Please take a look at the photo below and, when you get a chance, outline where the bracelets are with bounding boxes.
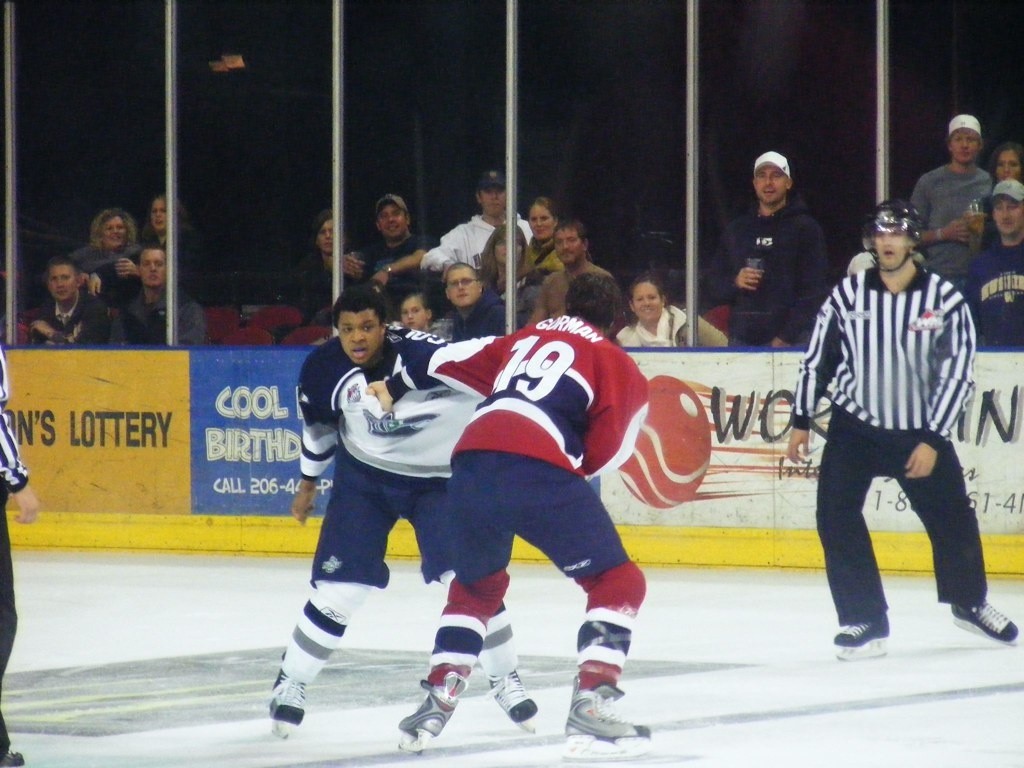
[386,263,393,281]
[936,228,944,240]
[45,329,52,338]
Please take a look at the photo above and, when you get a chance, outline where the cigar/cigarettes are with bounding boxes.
[755,263,773,274]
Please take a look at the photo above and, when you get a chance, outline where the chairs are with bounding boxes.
[202,306,243,342]
[222,326,276,347]
[247,305,304,339]
[702,305,729,334]
[280,325,332,345]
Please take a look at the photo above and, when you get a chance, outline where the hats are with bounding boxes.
[991,179,1024,202]
[376,194,409,216]
[753,150,791,179]
[477,169,507,191]
[949,114,981,136]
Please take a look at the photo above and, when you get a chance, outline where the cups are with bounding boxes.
[966,199,984,228]
[746,258,762,288]
[115,259,128,279]
[349,252,364,261]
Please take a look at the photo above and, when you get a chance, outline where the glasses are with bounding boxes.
[446,278,479,287]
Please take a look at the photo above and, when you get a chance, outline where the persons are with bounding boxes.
[29,190,209,349]
[295,171,728,349]
[0,345,39,768]
[964,178,1024,347]
[910,114,1024,296]
[261,284,538,739]
[398,272,651,761]
[788,198,1018,646]
[711,151,826,347]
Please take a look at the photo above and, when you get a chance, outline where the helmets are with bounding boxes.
[869,197,922,240]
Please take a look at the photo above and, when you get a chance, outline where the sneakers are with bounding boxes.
[834,612,890,662]
[489,670,540,734]
[560,675,653,764]
[0,750,26,768]
[950,600,1020,647]
[269,668,306,740]
[397,670,469,756]
